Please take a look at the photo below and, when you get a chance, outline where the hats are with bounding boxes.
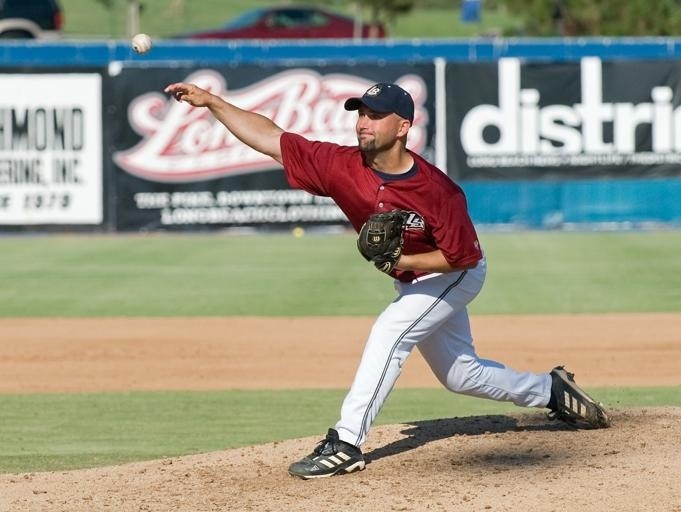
[344,82,414,120]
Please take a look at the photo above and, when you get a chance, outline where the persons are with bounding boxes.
[163,82,610,480]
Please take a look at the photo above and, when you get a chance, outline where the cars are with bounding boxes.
[0,1,64,39]
[165,5,388,39]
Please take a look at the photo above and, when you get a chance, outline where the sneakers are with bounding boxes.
[548,365,609,426]
[288,426,366,479]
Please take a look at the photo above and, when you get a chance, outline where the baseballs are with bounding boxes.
[132,34,152,54]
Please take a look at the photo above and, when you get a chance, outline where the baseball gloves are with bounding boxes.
[356,209,410,275]
